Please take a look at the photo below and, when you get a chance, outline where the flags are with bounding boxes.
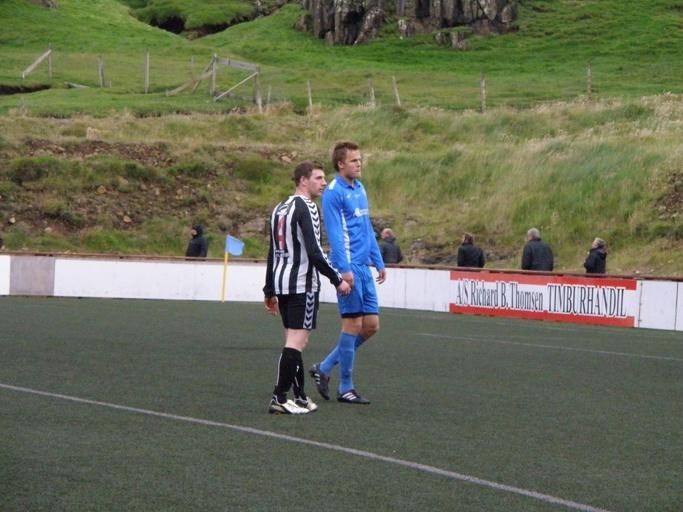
[225,235,245,257]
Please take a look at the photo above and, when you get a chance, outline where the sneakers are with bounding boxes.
[337,389,371,405]
[308,363,331,401]
[293,395,318,412]
[268,394,310,414]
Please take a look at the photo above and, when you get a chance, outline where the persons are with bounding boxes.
[309,140,386,404]
[378,228,401,264]
[459,231,485,272]
[264,160,351,414]
[584,236,606,272]
[521,227,554,271]
[184,224,208,261]
